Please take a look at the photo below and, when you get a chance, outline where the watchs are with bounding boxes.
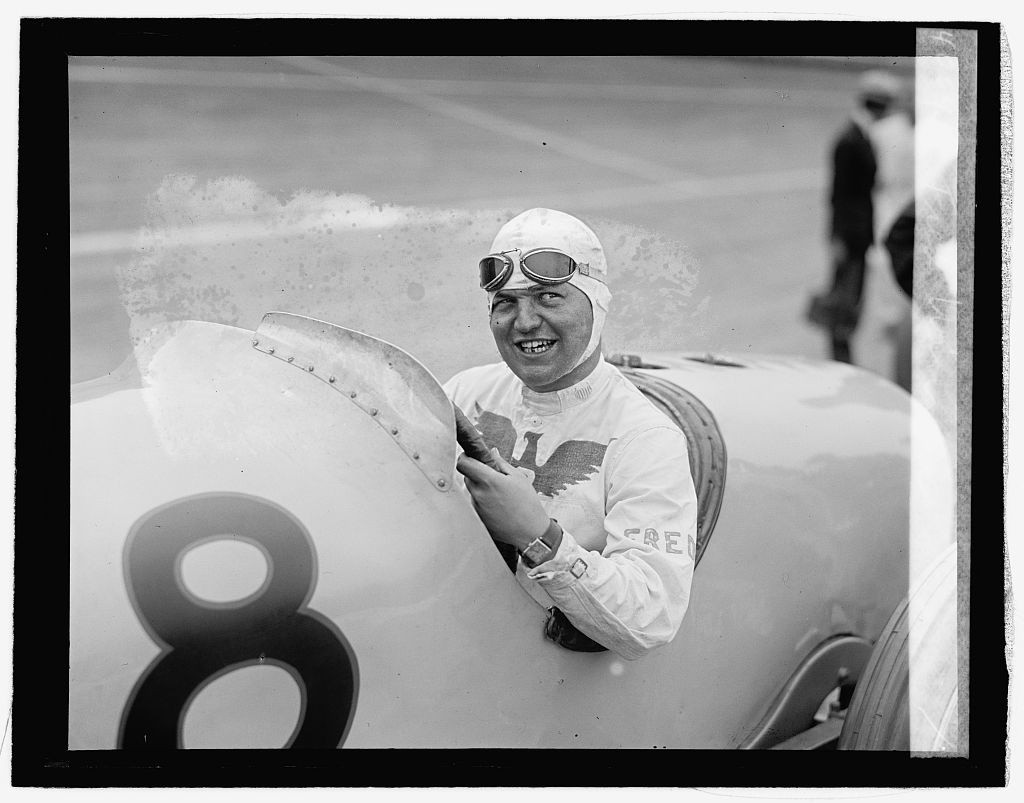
[521,519,569,575]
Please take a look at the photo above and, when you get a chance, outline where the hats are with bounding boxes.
[485,207,612,368]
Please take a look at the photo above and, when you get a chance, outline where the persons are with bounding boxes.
[809,69,896,367]
[356,208,703,659]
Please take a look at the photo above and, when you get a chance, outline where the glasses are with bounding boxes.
[479,247,606,293]
